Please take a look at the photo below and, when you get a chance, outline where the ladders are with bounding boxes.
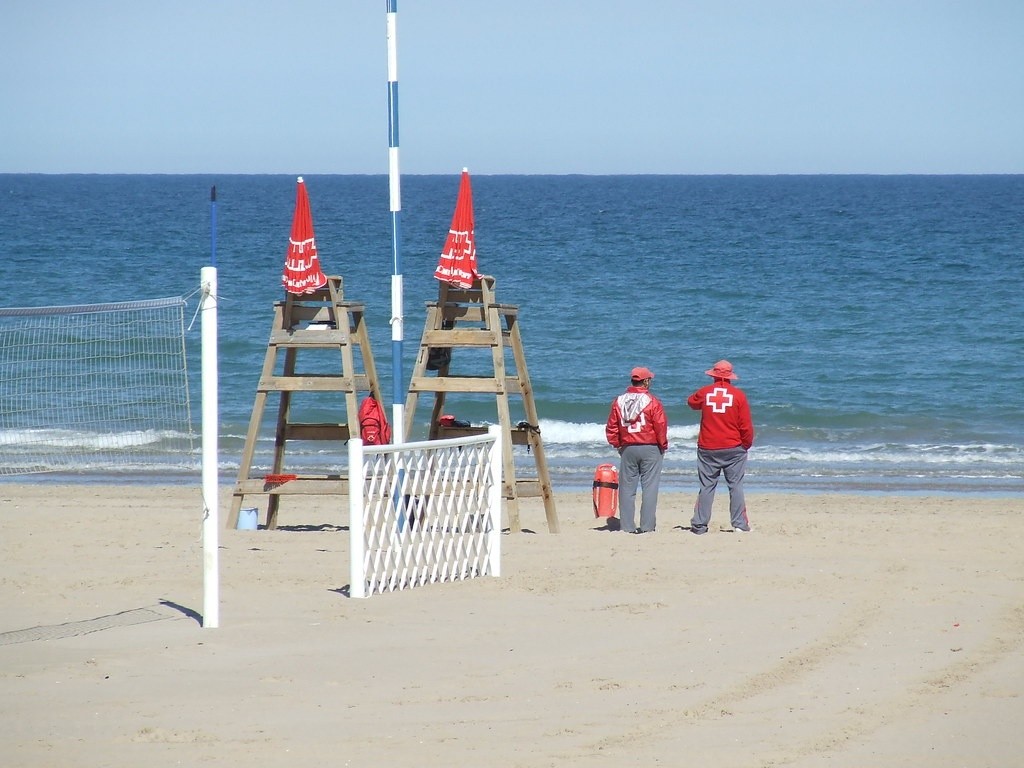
[391,302,561,544]
[225,300,402,529]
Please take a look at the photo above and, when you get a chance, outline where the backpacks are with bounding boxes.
[358,391,391,445]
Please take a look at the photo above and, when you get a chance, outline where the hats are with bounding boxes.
[631,367,654,380]
[705,360,736,379]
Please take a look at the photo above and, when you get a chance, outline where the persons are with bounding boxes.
[606,367,668,533]
[688,358,755,536]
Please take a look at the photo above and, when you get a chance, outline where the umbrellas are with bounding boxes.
[282,176,326,296]
[434,166,478,288]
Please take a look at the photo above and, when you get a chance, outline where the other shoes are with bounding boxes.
[735,528,743,532]
